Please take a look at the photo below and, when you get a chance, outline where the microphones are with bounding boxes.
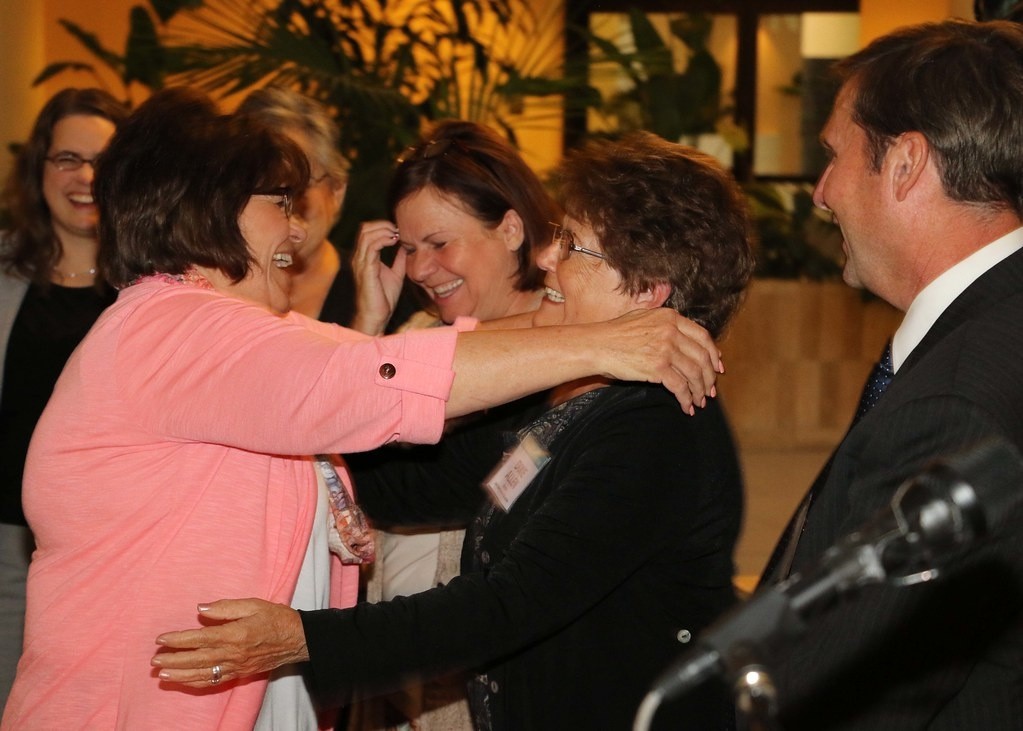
[649,435,1023,703]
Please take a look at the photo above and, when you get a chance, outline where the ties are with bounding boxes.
[787,335,894,580]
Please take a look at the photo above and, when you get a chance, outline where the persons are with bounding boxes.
[0,88,725,731]
[1,88,128,716]
[151,132,751,731]
[729,21,1023,731]
[235,84,423,731]
[351,122,562,731]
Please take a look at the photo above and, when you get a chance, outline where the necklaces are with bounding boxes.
[54,268,96,276]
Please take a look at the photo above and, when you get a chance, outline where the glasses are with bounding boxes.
[252,182,293,220]
[545,220,607,261]
[45,151,102,172]
[386,136,498,182]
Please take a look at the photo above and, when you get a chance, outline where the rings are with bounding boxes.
[212,666,221,684]
